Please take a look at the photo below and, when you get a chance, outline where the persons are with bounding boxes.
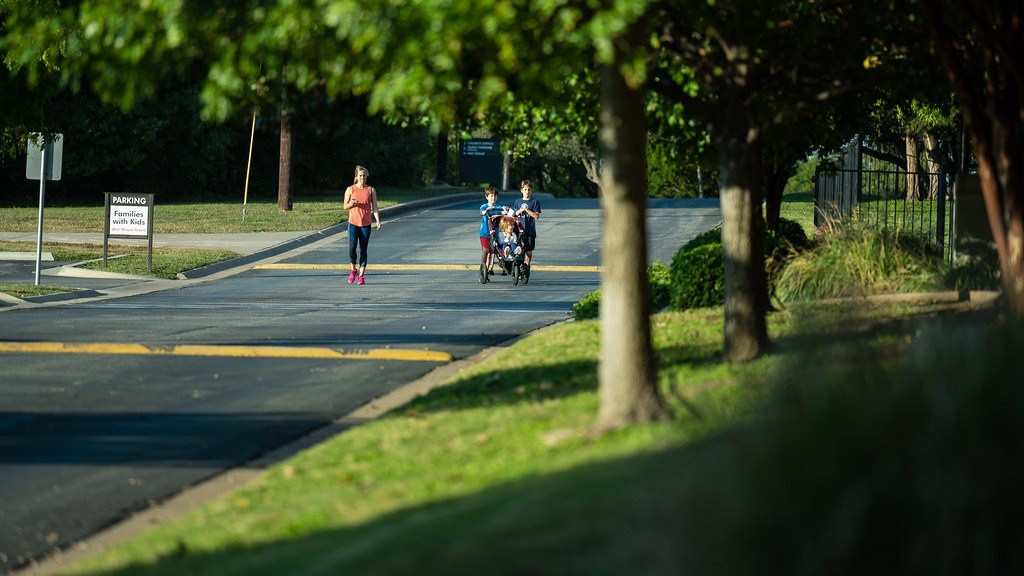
[342,165,382,284]
[475,180,542,283]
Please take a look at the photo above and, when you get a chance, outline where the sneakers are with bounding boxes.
[348,269,357,283]
[358,275,365,284]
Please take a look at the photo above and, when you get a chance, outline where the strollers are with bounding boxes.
[479,206,531,286]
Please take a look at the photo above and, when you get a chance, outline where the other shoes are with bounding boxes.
[504,246,513,260]
[488,268,494,275]
[513,246,522,259]
